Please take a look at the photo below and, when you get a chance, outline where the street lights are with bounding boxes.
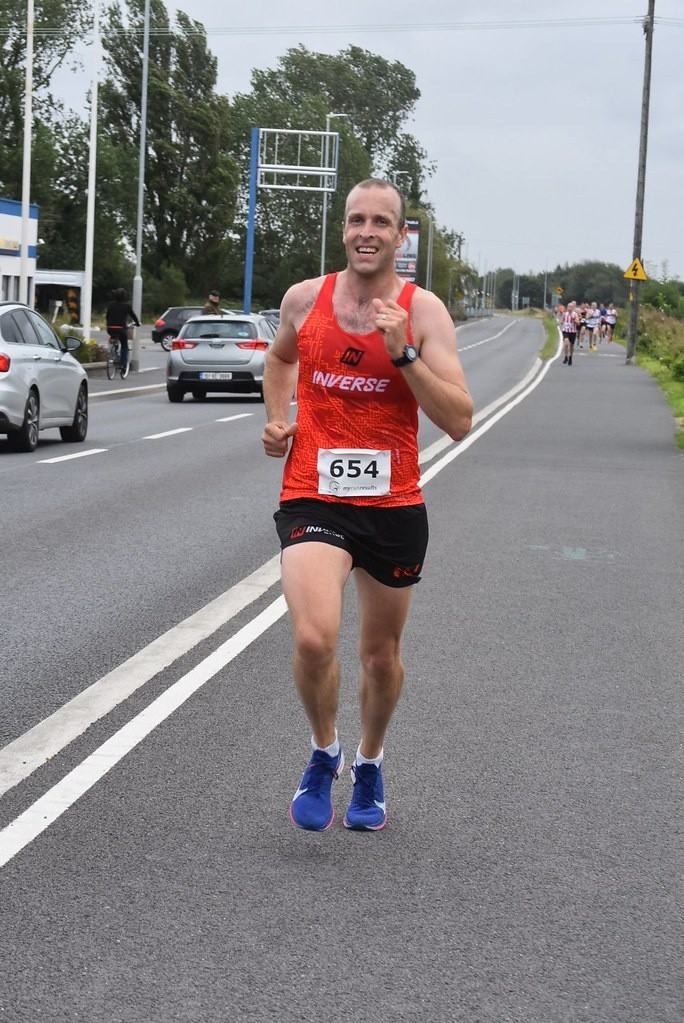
[392,169,410,185]
[319,110,354,277]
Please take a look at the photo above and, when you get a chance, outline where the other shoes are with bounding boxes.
[563,358,568,363]
[607,337,612,344]
[577,341,583,349]
[569,360,572,365]
[594,345,597,350]
[120,366,126,373]
[589,348,593,353]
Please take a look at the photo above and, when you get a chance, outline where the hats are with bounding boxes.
[209,290,220,297]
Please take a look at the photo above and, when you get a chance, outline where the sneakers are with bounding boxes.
[288,744,344,832]
[342,759,387,831]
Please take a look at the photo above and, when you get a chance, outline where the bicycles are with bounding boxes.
[106,323,141,381]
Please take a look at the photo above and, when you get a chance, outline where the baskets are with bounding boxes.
[126,326,136,340]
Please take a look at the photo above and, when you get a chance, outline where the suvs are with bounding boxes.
[0,300,90,454]
[150,305,240,352]
[164,315,296,403]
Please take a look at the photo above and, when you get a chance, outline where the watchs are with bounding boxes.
[391,344,417,367]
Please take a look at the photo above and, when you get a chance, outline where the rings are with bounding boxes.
[383,315,386,319]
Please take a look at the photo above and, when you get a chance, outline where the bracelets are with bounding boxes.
[573,318,575,320]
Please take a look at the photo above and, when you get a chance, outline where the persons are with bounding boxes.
[261,178,474,834]
[203,291,222,316]
[107,290,140,372]
[554,301,618,366]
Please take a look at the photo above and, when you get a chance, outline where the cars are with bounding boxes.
[229,308,280,330]
